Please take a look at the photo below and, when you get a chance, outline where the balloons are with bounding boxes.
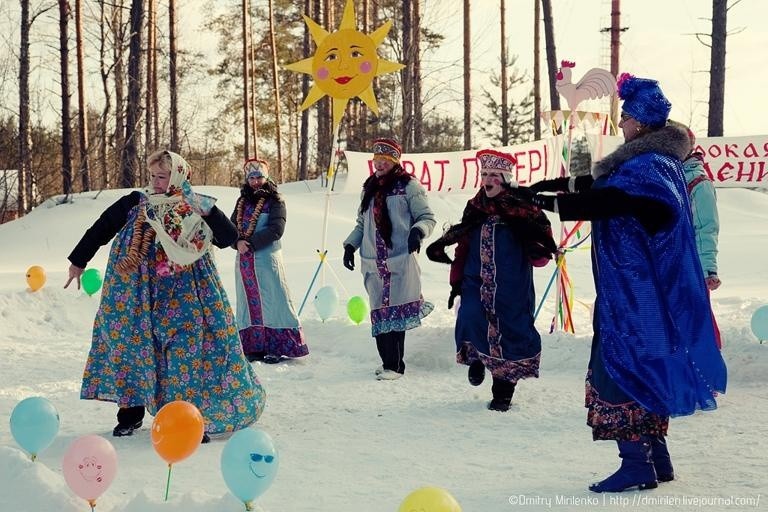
[25,265,47,293]
[312,286,339,320]
[749,305,767,342]
[346,295,366,322]
[149,400,205,463]
[9,396,59,457]
[398,486,463,512]
[80,268,104,300]
[220,428,280,503]
[64,435,119,501]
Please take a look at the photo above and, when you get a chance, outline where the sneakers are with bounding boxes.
[261,353,281,363]
[374,360,405,379]
[468,361,485,384]
[490,380,515,410]
[111,418,144,435]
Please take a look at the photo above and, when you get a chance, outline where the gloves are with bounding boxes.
[407,227,424,254]
[498,172,577,213]
[343,244,356,271]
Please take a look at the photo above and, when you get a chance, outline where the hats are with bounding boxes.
[371,138,403,167]
[476,149,518,184]
[616,73,672,128]
[243,159,271,180]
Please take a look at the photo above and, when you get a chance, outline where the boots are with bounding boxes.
[588,435,674,492]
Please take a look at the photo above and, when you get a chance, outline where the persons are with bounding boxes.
[501,76,728,492]
[342,138,437,381]
[231,158,309,364]
[669,121,724,353]
[63,150,251,442]
[424,147,558,410]
